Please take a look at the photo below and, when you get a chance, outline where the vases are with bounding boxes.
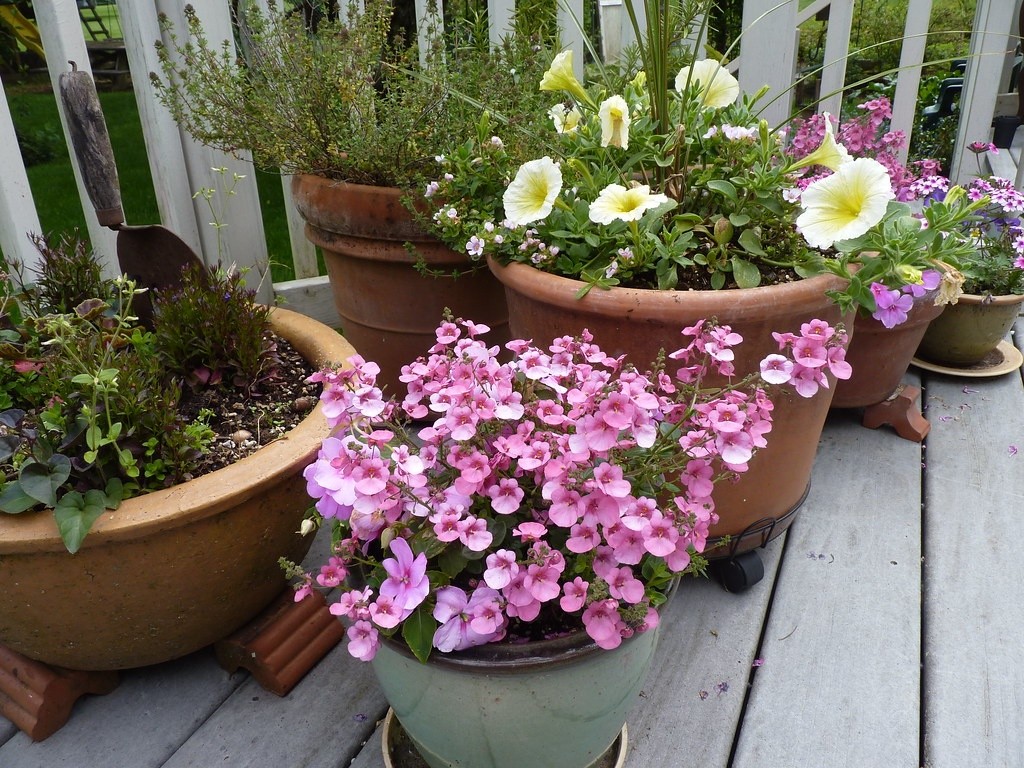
[292,155,514,426]
[830,290,945,442]
[909,293,1024,377]
[486,251,883,596]
[0,303,360,743]
[340,574,683,768]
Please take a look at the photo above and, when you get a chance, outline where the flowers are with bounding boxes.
[148,0,572,184]
[0,166,293,553]
[377,0,1024,329]
[275,303,852,661]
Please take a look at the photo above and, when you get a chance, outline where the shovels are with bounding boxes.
[56,59,212,294]
